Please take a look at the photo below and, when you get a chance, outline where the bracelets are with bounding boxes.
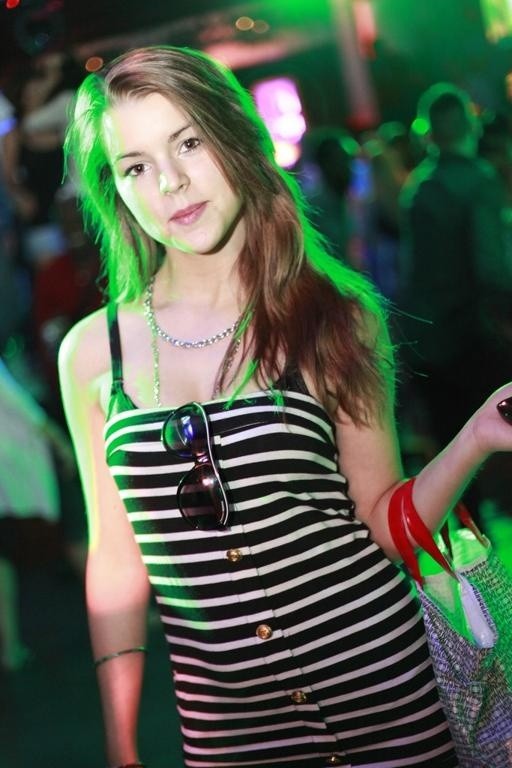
[93,646,151,669]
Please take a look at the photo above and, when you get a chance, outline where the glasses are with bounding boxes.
[162,400,229,531]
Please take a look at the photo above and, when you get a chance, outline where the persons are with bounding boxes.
[56,45,511,768]
[0,360,72,664]
[0,28,512,511]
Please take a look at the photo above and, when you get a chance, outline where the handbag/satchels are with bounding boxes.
[388,478,512,767]
